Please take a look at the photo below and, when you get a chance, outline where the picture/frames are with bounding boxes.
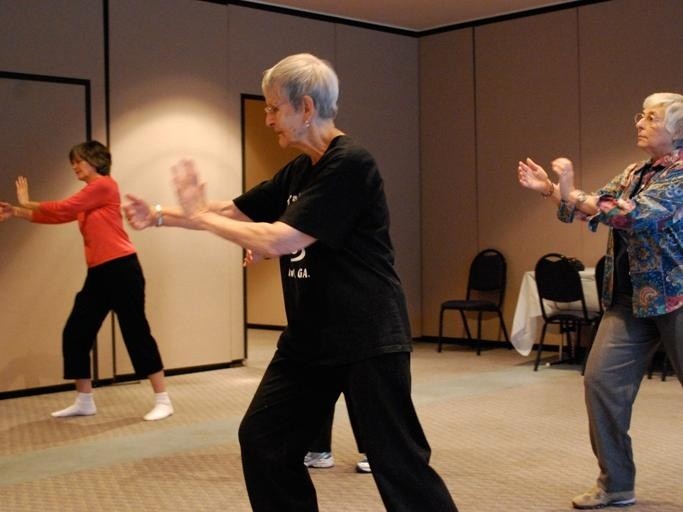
[510,267,606,368]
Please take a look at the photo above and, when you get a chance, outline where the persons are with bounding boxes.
[515,89,683,511]
[243,246,373,475]
[1,140,176,421]
[122,49,461,512]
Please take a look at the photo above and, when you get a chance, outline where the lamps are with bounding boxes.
[534,253,600,377]
[595,255,653,380]
[437,249,511,356]
[661,353,674,381]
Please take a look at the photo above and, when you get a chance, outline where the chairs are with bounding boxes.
[355,454,372,473]
[572,484,637,510]
[304,451,334,468]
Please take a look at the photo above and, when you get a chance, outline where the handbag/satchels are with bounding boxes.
[155,205,164,226]
[541,183,554,198]
[575,190,586,210]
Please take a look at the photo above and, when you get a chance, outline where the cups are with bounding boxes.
[634,112,665,125]
